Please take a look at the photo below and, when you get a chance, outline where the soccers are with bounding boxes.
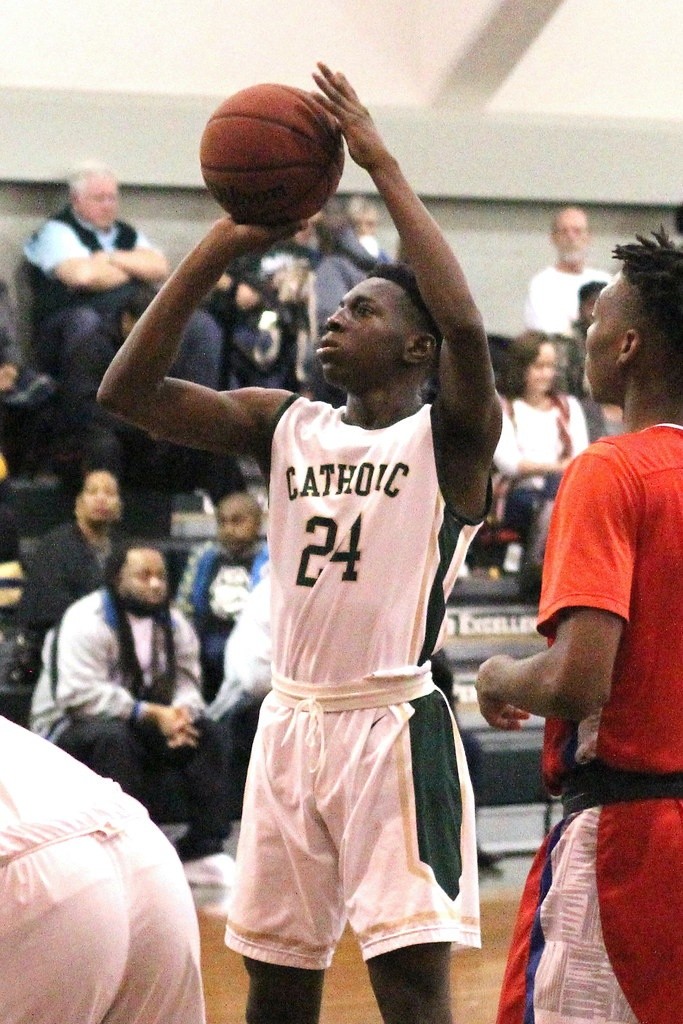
[199,82,346,224]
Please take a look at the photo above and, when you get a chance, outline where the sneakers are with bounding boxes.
[181,851,234,885]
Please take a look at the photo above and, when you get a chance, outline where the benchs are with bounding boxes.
[0,469,556,823]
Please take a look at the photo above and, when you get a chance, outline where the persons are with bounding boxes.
[485,206,629,606]
[475,222,683,1024]
[0,159,388,887]
[0,715,207,1024]
[97,62,502,1024]
